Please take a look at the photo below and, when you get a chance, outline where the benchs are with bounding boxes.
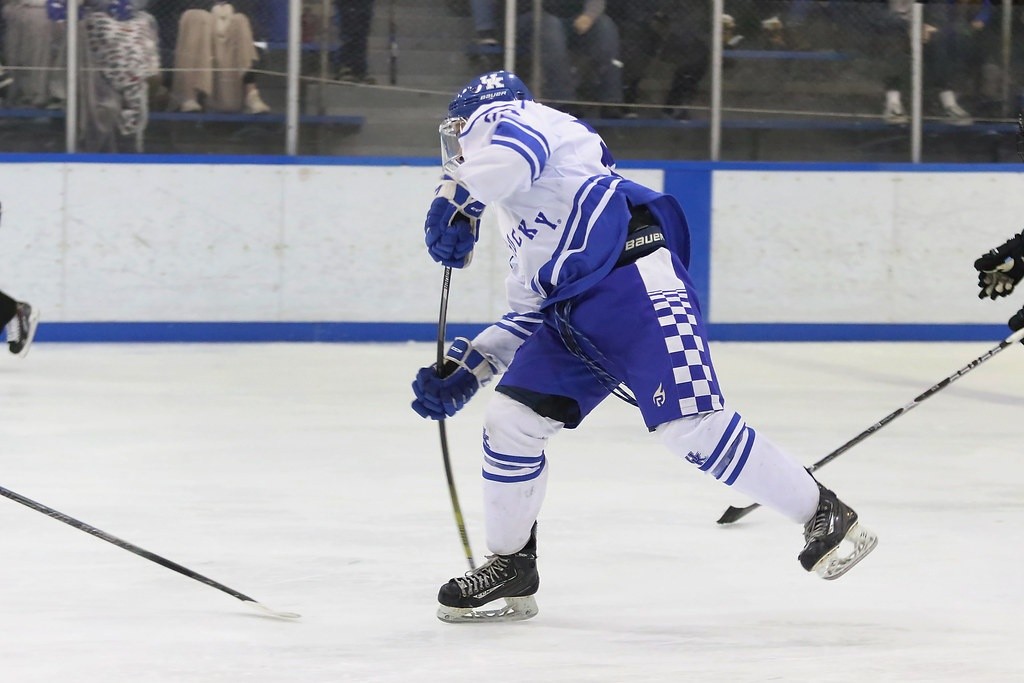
[467,0,1024,164]
[0,0,375,157]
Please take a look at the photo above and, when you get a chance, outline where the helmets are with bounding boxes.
[445,70,535,120]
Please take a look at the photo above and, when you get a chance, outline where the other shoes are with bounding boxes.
[933,104,973,127]
[882,102,909,128]
[359,74,378,86]
[180,99,202,112]
[600,100,621,118]
[621,93,638,118]
[661,99,691,123]
[243,88,271,115]
[334,65,356,82]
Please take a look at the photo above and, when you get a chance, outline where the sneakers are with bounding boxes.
[6,301,41,358]
[799,466,878,581]
[436,520,540,623]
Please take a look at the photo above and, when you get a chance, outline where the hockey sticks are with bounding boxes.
[715,326,1024,527]
[430,212,478,572]
[1,482,306,623]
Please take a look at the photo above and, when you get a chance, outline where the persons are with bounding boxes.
[468,0,1024,126]
[410,70,878,623]
[0,291,41,360]
[974,229,1024,346]
[0,0,375,153]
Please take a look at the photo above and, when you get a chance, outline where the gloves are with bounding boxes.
[411,336,495,421]
[424,170,488,270]
[973,233,1023,300]
